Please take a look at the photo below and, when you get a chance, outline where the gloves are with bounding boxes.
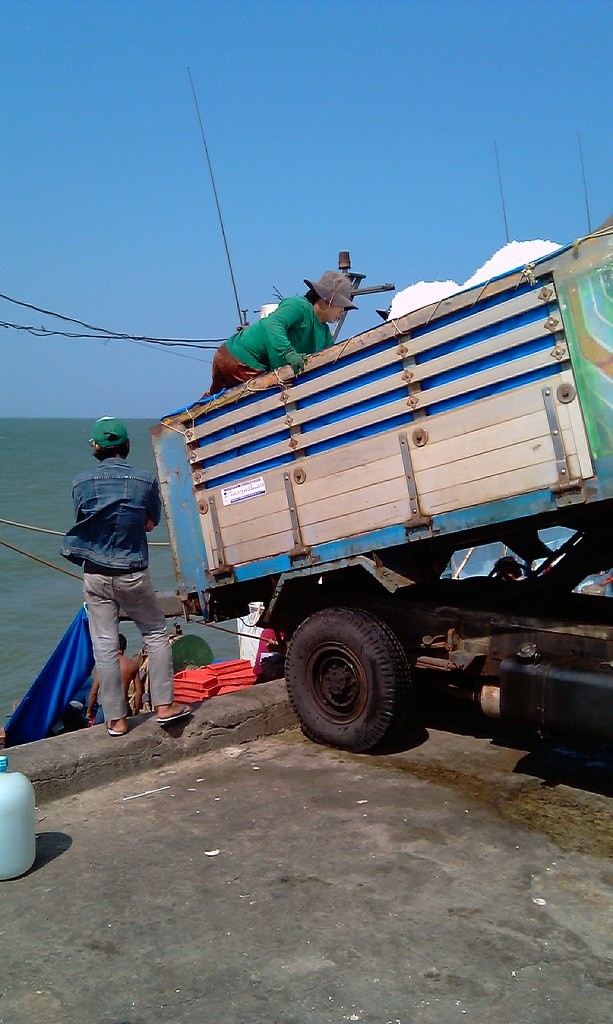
[284,351,307,374]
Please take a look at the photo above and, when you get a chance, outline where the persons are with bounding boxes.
[201,270,359,399]
[87,633,143,724]
[58,417,193,737]
[495,556,527,581]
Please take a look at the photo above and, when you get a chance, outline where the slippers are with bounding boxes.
[157,704,190,722]
[104,721,123,734]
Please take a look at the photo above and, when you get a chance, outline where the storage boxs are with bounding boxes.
[174,659,257,704]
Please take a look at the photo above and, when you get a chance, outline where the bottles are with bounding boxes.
[0,755,35,880]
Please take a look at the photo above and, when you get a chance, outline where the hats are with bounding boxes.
[303,270,359,310]
[88,416,128,448]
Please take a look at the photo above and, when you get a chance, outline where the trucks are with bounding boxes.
[150,211,613,756]
